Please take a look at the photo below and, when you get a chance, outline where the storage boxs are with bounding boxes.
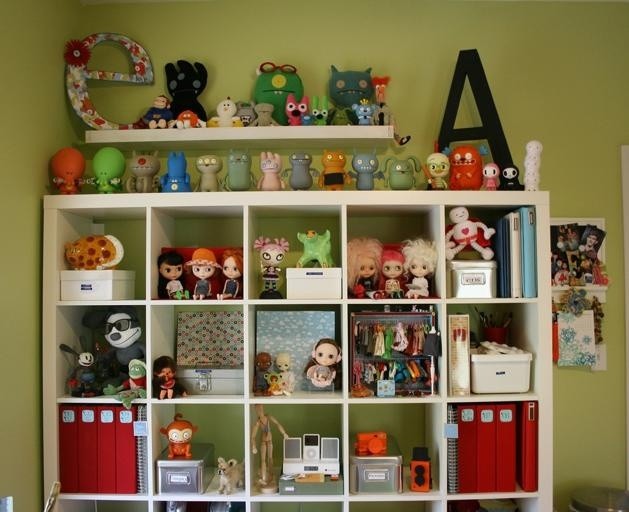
[155,441,216,494]
[464,350,532,396]
[56,270,138,304]
[351,433,406,497]
[283,264,344,302]
[448,259,496,301]
[173,364,246,394]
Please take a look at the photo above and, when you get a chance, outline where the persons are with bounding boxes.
[347,236,437,299]
[254,338,342,395]
[251,404,289,483]
[157,247,242,301]
[550,223,601,283]
[254,236,290,293]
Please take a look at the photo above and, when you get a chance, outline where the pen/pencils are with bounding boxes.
[44,481,61,512]
[474,306,513,328]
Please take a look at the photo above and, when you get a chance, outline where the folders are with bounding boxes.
[58,405,138,494]
[457,404,516,493]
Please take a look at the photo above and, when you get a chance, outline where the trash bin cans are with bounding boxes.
[568,487,629,512]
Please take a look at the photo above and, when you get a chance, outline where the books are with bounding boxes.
[447,406,457,495]
[137,405,148,494]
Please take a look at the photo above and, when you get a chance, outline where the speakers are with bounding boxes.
[282,437,302,464]
[321,438,339,462]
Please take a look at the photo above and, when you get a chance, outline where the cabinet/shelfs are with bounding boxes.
[44,191,554,512]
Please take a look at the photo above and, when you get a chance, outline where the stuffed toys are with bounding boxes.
[152,356,187,400]
[60,307,146,408]
